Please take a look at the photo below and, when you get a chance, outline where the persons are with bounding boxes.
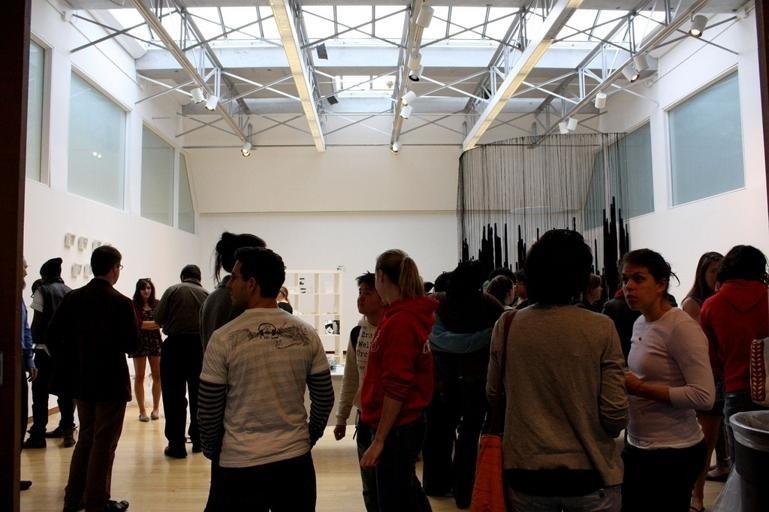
[680,252,728,511]
[20,257,38,492]
[699,245,769,466]
[199,231,266,353]
[153,264,209,459]
[126,279,162,422]
[335,271,384,512]
[196,247,334,512]
[23,257,76,448]
[59,246,136,512]
[486,228,629,512]
[575,273,603,313]
[423,259,526,512]
[622,248,717,511]
[277,286,293,315]
[356,247,438,512]
[29,278,76,439]
[602,257,678,369]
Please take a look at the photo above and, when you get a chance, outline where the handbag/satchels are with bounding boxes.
[468,306,517,512]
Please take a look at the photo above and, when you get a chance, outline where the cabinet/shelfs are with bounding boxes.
[278,269,343,367]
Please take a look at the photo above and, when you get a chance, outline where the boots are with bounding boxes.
[24,435,46,449]
[65,428,75,447]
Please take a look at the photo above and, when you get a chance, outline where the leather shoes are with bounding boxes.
[138,414,149,421]
[19,480,31,489]
[112,498,128,512]
[163,446,188,458]
[46,426,69,438]
[152,412,159,419]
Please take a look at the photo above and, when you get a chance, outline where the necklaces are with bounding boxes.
[638,304,672,341]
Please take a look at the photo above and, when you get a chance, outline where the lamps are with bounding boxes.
[400,103,412,119]
[309,43,328,59]
[320,96,339,104]
[416,0,433,28]
[621,65,638,83]
[633,54,649,72]
[401,87,416,104]
[408,45,422,70]
[206,92,218,112]
[391,140,401,153]
[566,116,577,131]
[190,86,204,103]
[408,64,423,82]
[689,11,708,38]
[595,89,607,108]
[242,142,251,157]
[558,119,568,134]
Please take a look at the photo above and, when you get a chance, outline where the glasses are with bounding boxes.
[119,264,123,270]
